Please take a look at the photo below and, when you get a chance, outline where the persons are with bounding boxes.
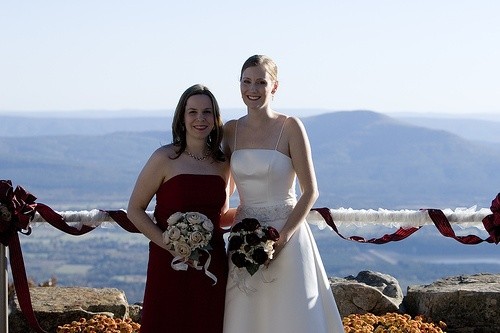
[220,55,348,333]
[127,84,242,333]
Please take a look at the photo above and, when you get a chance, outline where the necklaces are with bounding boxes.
[183,141,211,161]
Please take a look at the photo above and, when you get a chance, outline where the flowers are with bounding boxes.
[162,211,215,271]
[226,218,281,277]
